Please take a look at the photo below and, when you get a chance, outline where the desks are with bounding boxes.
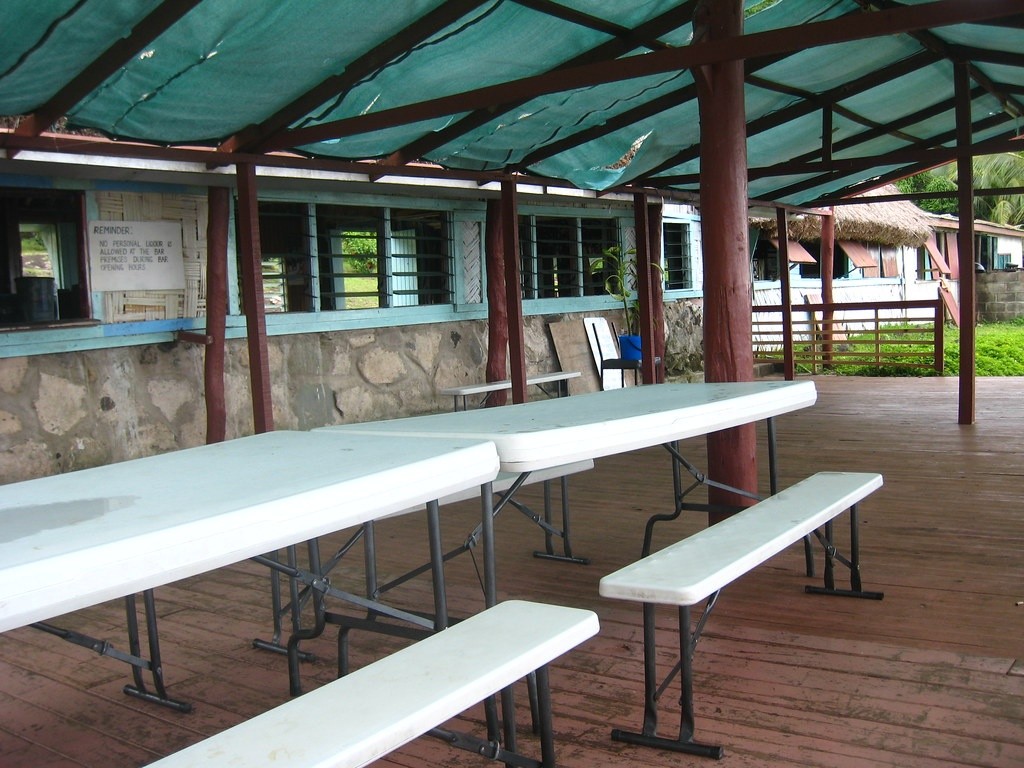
[1,381,818,768]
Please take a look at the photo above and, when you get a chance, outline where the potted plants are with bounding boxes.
[588,245,664,359]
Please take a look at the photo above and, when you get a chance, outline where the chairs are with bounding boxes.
[592,322,661,392]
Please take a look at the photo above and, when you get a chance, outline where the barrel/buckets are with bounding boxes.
[14,277,60,323]
[618,335,642,360]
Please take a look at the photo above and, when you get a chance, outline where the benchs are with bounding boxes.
[253,458,594,714]
[599,470,884,760]
[441,371,582,413]
[143,600,601,768]
